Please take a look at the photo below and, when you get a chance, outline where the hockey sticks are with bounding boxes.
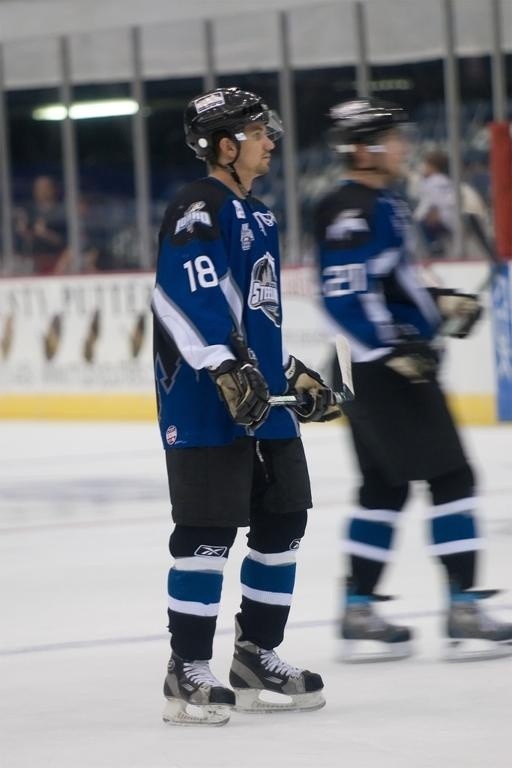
[386,212,502,385]
[265,333,356,408]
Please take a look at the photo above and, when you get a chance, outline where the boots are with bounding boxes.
[447,570,512,642]
[341,575,411,643]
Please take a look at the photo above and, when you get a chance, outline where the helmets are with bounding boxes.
[321,95,419,160]
[183,87,286,160]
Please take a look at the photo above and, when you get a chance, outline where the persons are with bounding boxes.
[409,150,493,261]
[13,175,69,277]
[312,95,512,644]
[148,84,346,705]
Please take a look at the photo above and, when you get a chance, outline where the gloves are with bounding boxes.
[284,355,344,423]
[382,340,441,384]
[432,289,482,337]
[207,329,272,433]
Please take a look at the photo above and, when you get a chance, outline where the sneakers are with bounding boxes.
[164,649,237,707]
[229,612,324,697]
